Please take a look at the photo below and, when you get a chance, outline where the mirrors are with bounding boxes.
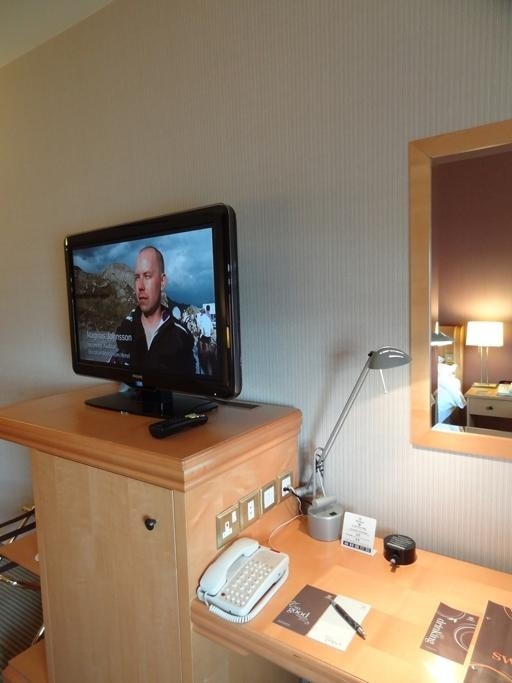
[408,119,512,462]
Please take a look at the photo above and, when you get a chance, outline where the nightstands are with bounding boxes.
[464,382,512,428]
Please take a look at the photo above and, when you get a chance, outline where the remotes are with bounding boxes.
[149,413,208,438]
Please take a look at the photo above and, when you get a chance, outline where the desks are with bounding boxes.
[0,382,302,683]
[191,516,512,683]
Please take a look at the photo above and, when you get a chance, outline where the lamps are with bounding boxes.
[464,320,504,388]
[293,347,410,542]
[430,333,453,346]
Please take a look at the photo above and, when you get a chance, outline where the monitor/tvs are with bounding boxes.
[62,202,243,421]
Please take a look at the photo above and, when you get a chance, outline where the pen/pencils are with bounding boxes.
[328,597,367,640]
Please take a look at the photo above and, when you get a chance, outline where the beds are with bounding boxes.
[439,324,467,424]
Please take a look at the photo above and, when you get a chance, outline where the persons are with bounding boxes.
[206,305,211,317]
[188,308,194,319]
[196,307,214,347]
[108,246,197,376]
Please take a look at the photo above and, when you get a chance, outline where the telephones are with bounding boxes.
[196,537,290,623]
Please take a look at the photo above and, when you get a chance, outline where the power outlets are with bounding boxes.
[216,470,293,549]
